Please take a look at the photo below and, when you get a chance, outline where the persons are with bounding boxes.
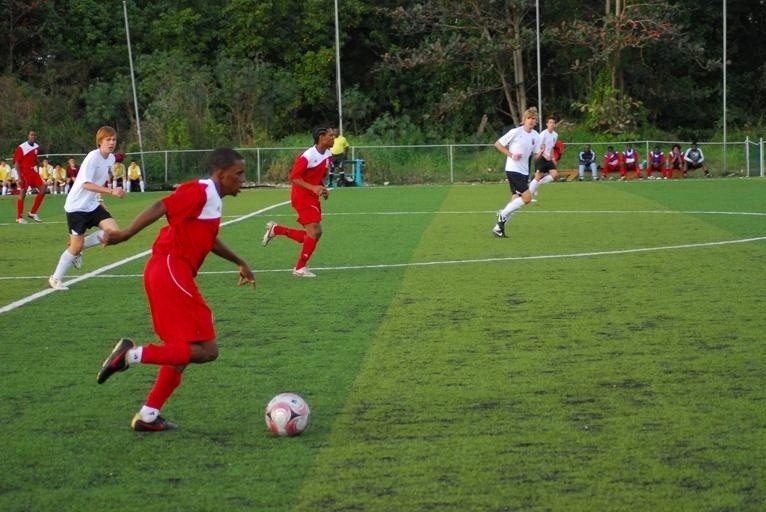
[491,107,544,239]
[325,128,348,187]
[46,125,127,292]
[620,143,642,181]
[666,144,684,180]
[646,146,667,180]
[527,115,560,202]
[683,144,711,178]
[0,155,143,196]
[13,130,47,224]
[601,146,624,180]
[260,122,334,278]
[578,144,598,181]
[97,145,257,432]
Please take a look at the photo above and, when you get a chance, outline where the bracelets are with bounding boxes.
[511,153,514,158]
[109,189,113,195]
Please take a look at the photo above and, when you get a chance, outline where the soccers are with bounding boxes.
[264,393,310,436]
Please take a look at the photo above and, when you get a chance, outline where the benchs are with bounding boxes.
[557,169,696,182]
[327,159,365,187]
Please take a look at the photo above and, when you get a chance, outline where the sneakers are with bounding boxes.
[491,208,506,238]
[48,274,69,290]
[16,217,28,225]
[578,172,711,181]
[66,239,82,270]
[96,336,137,385]
[261,220,278,247]
[130,411,179,433]
[27,211,42,222]
[292,266,317,278]
